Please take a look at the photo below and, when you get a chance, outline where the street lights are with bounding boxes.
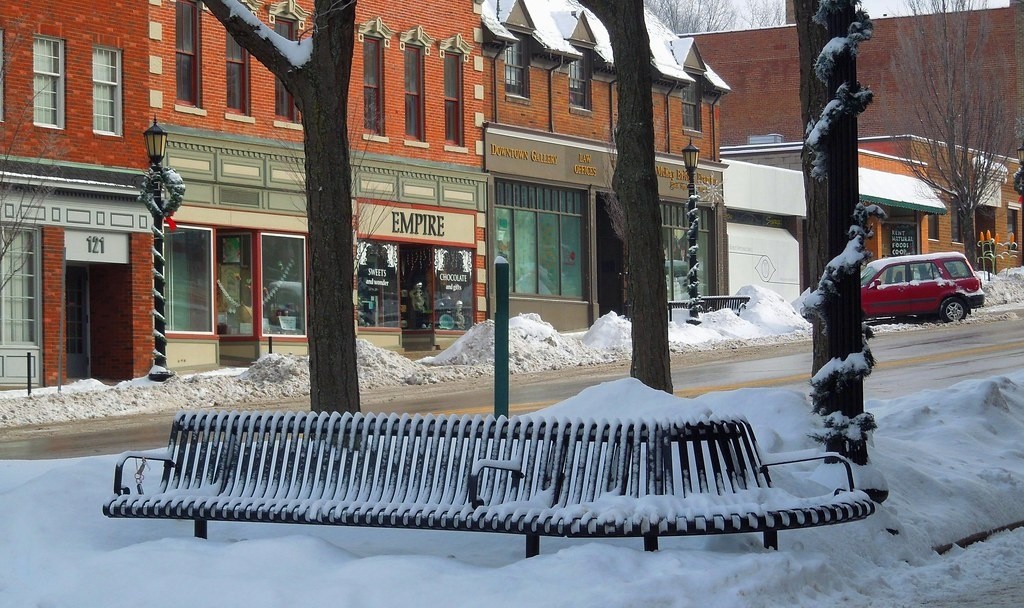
[1017,141,1024,265]
[141,113,172,382]
[682,136,701,318]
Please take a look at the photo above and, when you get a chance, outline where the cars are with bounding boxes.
[860,252,984,323]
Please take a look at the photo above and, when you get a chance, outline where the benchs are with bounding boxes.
[104,409,876,562]
